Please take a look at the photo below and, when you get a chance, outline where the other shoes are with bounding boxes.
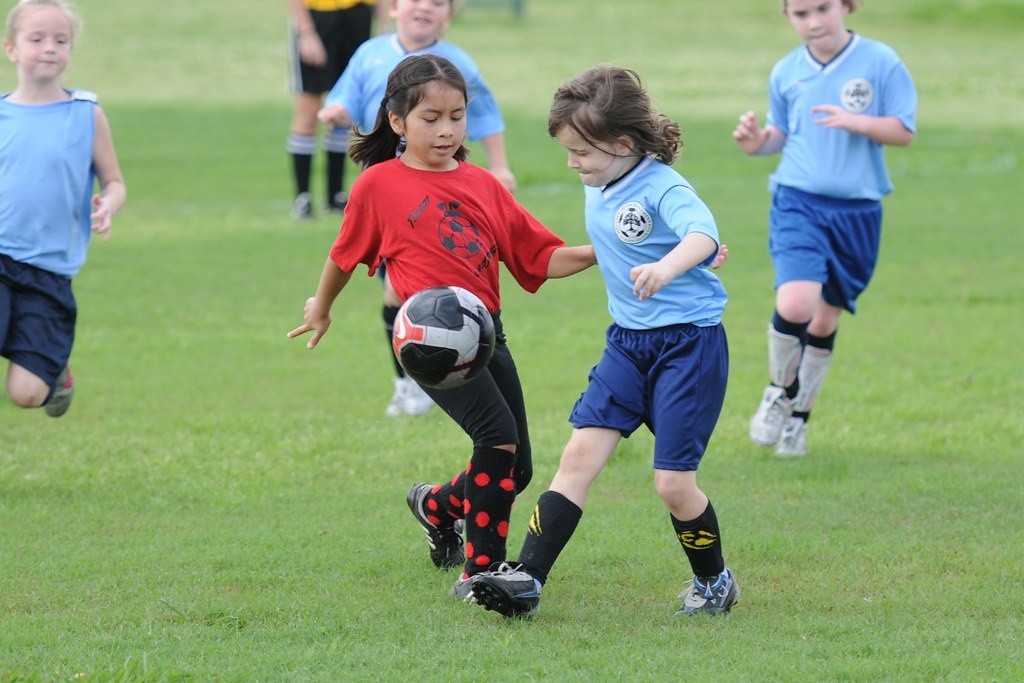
[290,190,316,219]
[328,191,351,216]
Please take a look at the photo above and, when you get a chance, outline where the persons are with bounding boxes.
[285,1,516,220]
[472,66,741,623]
[731,1,918,457]
[0,0,126,420]
[288,55,598,606]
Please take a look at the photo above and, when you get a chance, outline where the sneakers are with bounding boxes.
[775,411,808,458]
[384,376,437,420]
[448,571,492,608]
[673,567,740,618]
[750,384,799,448]
[470,563,544,624]
[406,481,465,571]
[43,363,75,418]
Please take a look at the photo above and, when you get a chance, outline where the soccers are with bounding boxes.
[391,285,497,390]
